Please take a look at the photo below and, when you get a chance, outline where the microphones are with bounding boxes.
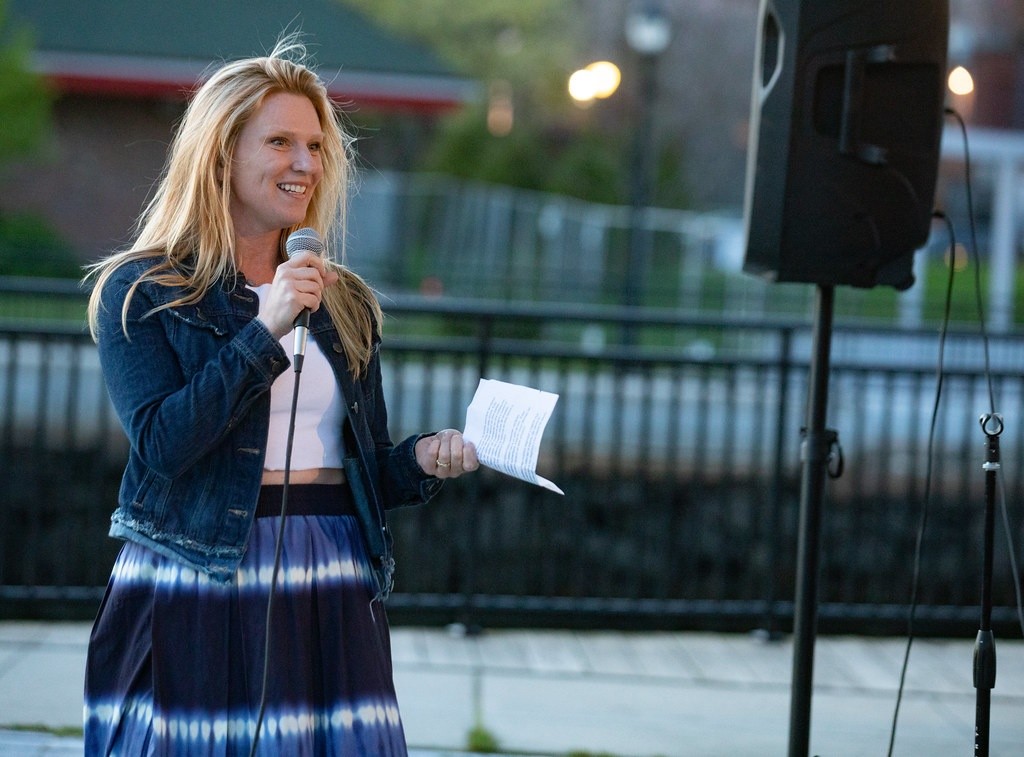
[285,228,325,372]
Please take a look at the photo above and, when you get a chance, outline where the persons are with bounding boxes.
[82,11,480,757]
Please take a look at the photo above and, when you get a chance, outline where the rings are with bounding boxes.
[436,460,451,467]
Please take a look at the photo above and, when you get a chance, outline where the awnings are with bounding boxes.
[27,51,461,118]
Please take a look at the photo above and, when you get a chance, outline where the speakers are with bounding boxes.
[740,0,951,290]
